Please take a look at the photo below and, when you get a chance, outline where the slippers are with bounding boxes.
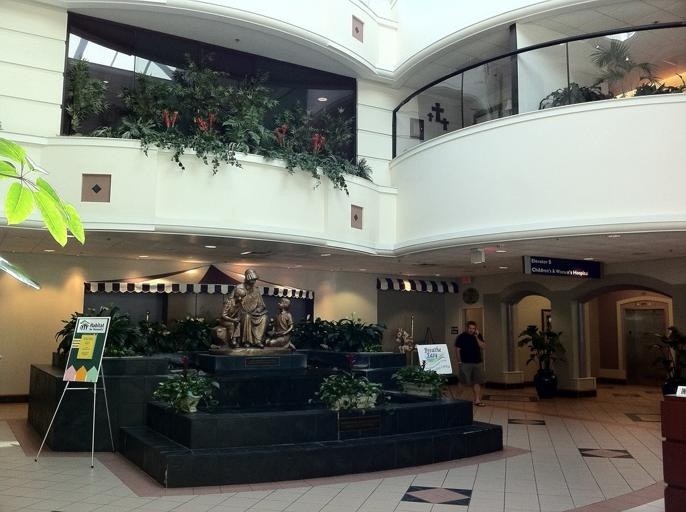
[475,402,486,407]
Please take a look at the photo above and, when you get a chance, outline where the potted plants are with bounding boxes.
[640,325,686,398]
[308,365,449,417]
[151,375,221,416]
[517,325,567,398]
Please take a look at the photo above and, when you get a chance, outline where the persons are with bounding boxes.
[263,297,295,349]
[231,269,268,351]
[222,286,246,350]
[451,321,488,408]
